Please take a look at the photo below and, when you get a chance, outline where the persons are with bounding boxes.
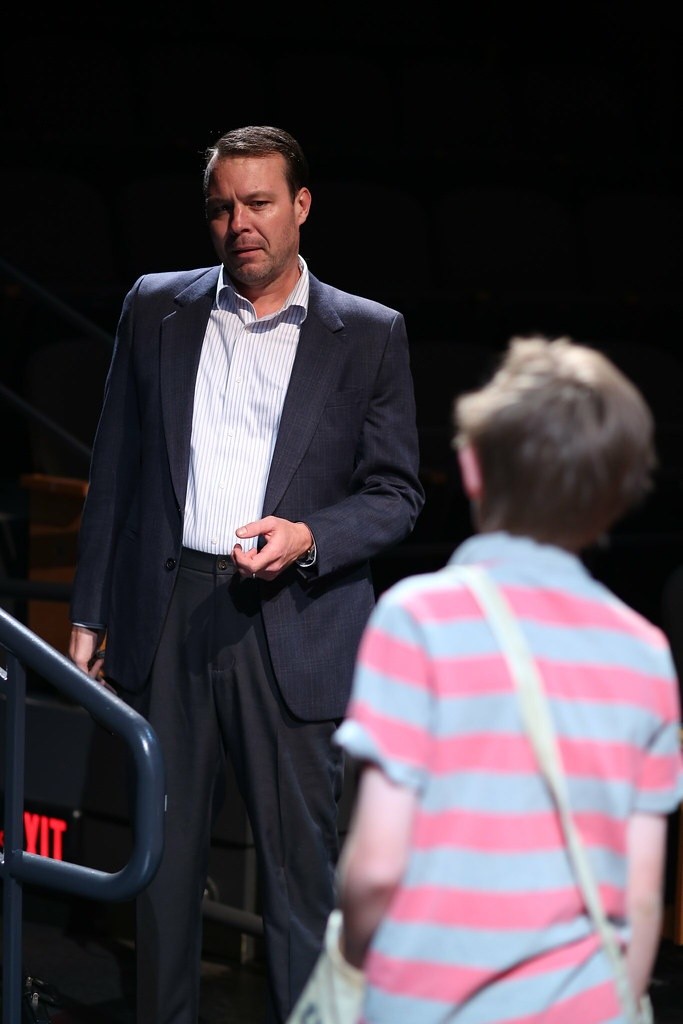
[67,127,423,1024]
[336,337,683,1024]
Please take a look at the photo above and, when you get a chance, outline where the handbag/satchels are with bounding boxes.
[284,909,366,1024]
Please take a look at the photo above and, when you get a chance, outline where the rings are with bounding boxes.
[253,573,256,578]
